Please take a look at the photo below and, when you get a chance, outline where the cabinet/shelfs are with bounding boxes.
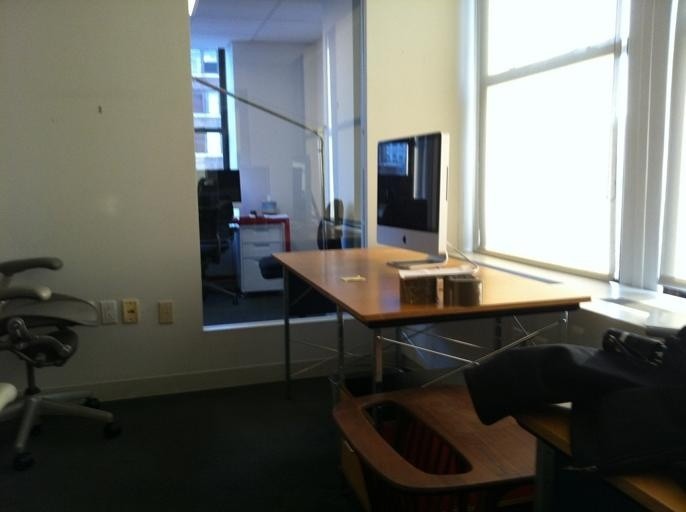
[232,213,288,299]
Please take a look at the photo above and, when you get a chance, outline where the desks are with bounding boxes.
[271,244,592,427]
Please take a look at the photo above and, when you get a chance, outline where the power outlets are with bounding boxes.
[99,298,138,325]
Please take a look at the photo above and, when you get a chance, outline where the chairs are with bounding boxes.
[0,257,121,471]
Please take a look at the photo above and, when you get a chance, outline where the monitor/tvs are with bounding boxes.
[376,130,449,270]
